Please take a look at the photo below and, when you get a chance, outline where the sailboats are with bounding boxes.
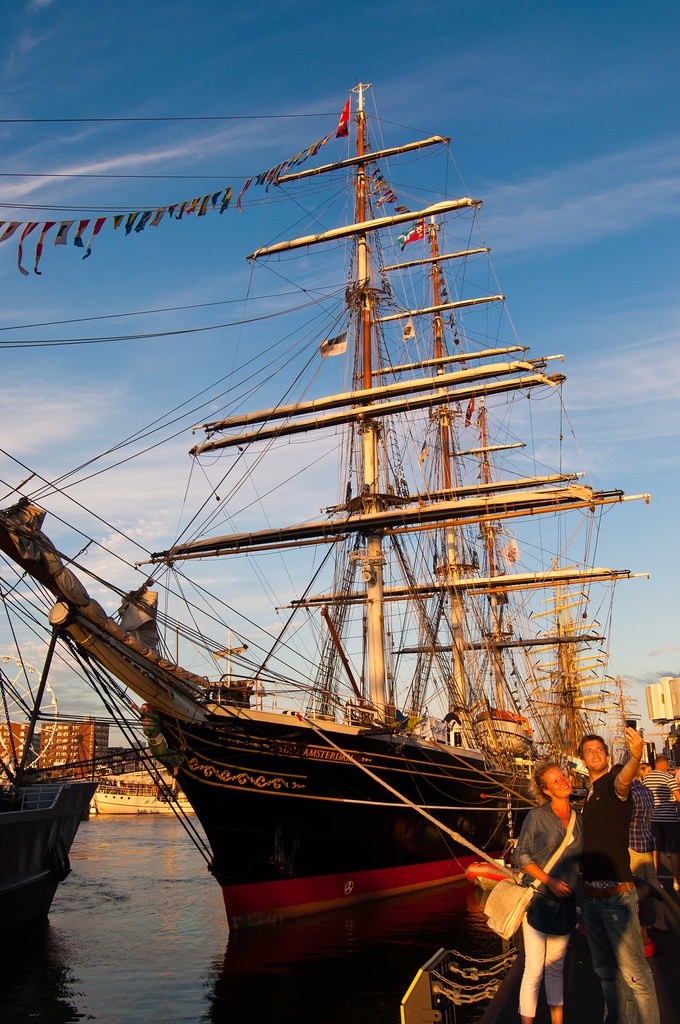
[0,82,652,932]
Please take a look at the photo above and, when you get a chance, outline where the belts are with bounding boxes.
[588,880,635,897]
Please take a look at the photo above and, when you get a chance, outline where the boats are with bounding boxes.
[0,777,100,948]
[84,769,205,816]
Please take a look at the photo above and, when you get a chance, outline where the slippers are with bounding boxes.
[648,922,672,932]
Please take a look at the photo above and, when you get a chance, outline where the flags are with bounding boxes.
[0,98,475,466]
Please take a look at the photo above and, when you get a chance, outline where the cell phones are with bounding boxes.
[626,720,637,737]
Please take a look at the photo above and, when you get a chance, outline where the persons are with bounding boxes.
[578,727,660,1024]
[515,763,584,1024]
[608,755,680,957]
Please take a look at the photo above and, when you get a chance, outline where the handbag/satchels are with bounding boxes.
[484,878,535,941]
